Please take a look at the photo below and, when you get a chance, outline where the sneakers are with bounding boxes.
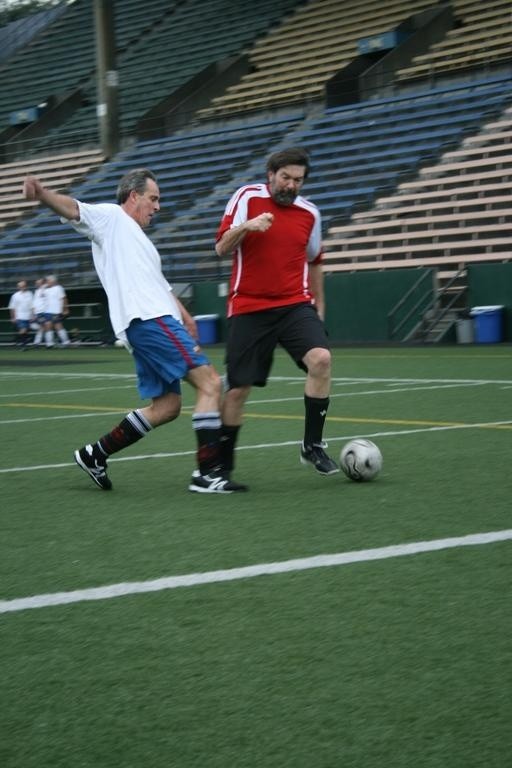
[300,438,340,475]
[188,463,249,494]
[74,443,112,490]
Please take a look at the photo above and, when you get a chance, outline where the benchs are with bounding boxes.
[0,0,509,288]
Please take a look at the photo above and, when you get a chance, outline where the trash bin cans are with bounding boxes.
[455,305,505,344]
[192,313,220,345]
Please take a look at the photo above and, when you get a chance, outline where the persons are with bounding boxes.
[7,274,72,352]
[214,146,342,476]
[22,166,250,495]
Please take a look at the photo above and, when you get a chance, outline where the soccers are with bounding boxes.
[338,438,384,481]
